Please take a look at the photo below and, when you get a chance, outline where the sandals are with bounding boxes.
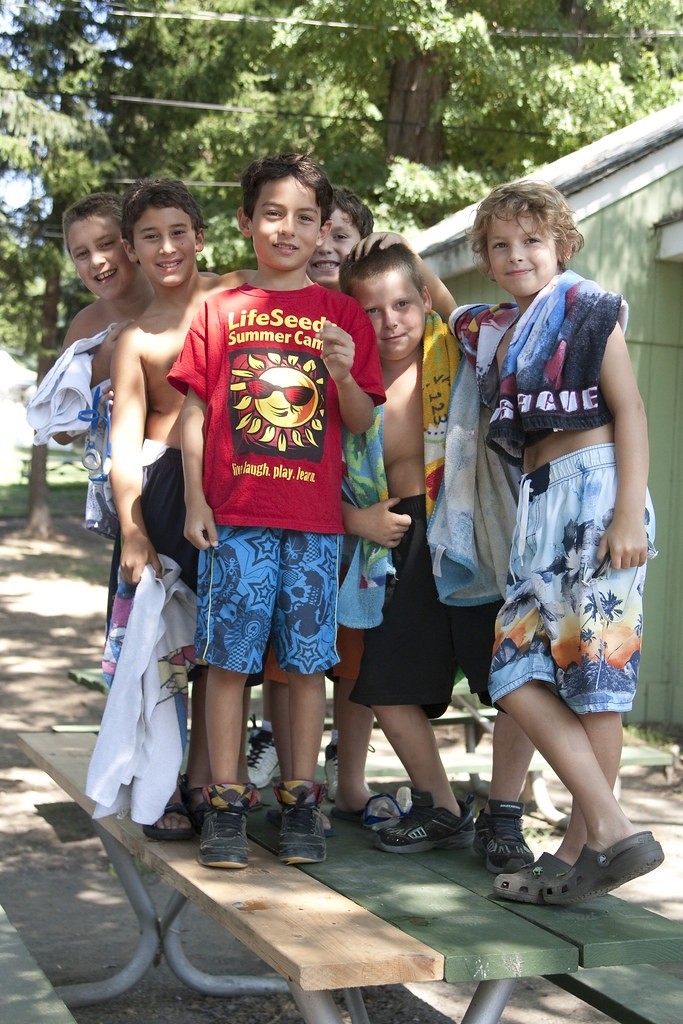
[185,787,212,833]
[142,771,195,840]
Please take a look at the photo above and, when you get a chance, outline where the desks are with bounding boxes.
[68,668,500,753]
[13,732,683,1024]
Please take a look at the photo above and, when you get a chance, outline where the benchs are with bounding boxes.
[540,963,683,1024]
[0,904,78,1024]
[322,707,499,754]
[310,744,674,831]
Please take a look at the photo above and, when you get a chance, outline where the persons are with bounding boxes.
[242,667,350,806]
[168,152,389,870]
[59,194,159,392]
[345,179,664,905]
[338,240,542,880]
[107,177,264,839]
[268,185,385,837]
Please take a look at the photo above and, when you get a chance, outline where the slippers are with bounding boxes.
[544,831,665,903]
[268,810,335,838]
[494,852,573,903]
[331,799,395,822]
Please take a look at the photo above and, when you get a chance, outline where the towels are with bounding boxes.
[332,305,523,628]
[445,268,632,466]
[87,551,199,825]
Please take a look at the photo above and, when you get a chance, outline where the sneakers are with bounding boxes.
[473,799,534,873]
[374,787,475,853]
[197,781,257,868]
[325,742,375,801]
[244,714,279,788]
[273,780,329,864]
